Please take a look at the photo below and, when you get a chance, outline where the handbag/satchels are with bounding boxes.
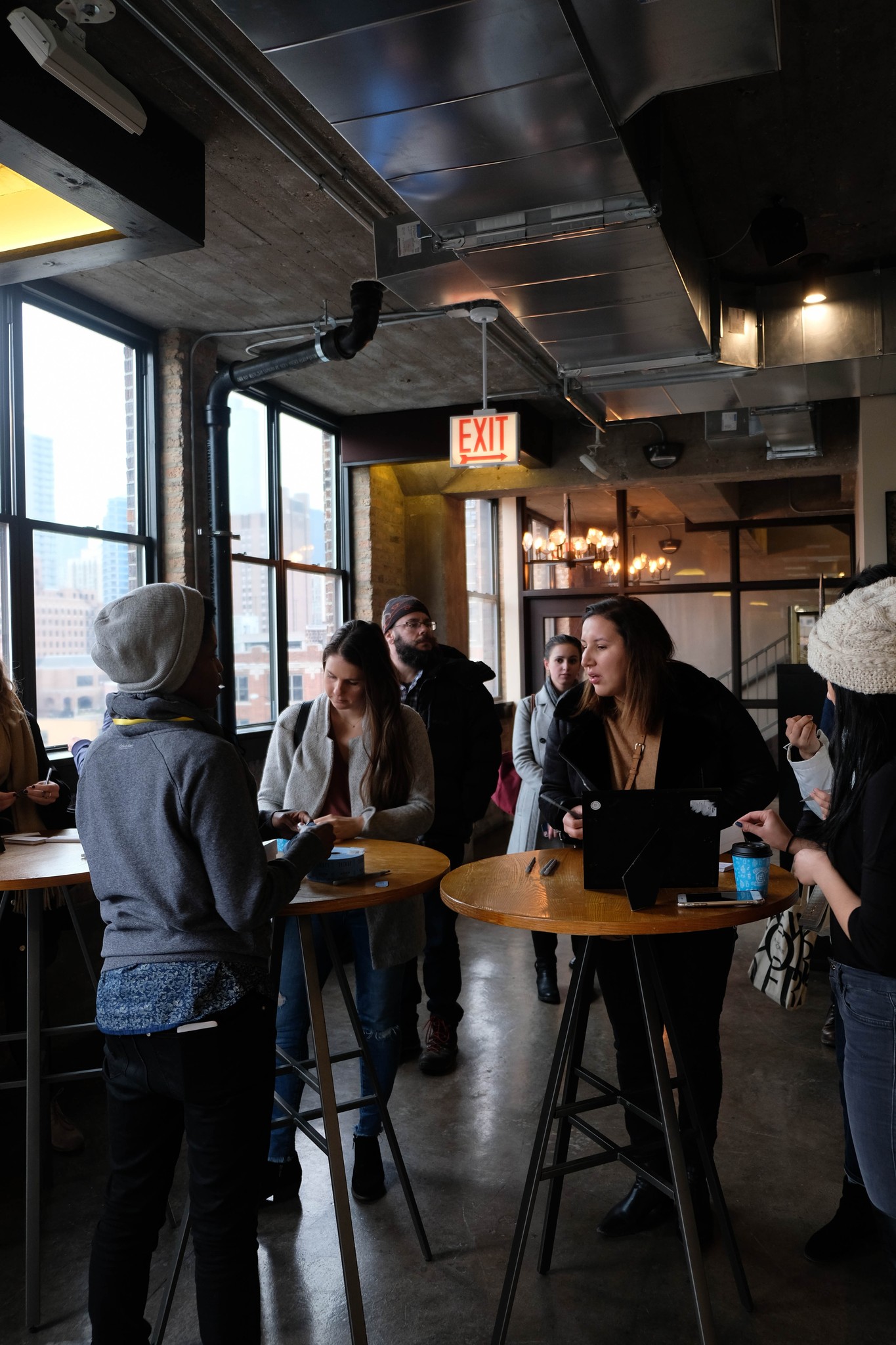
[489,750,526,815]
[747,901,816,1010]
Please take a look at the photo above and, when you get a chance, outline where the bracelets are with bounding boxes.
[785,835,797,853]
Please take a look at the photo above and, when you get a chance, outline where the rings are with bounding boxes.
[48,792,52,799]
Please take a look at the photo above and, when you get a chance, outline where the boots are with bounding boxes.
[537,966,562,1001]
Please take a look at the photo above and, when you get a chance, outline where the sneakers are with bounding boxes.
[400,1029,421,1054]
[419,1024,462,1073]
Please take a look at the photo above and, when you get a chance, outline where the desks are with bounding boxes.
[0,824,143,1338]
[438,844,802,1345]
[144,832,453,1345]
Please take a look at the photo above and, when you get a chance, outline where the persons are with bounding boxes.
[69,585,363,1345]
[535,590,778,1252]
[0,658,91,1153]
[784,563,896,1258]
[377,595,503,1076]
[731,570,896,1223]
[251,620,434,1203]
[491,634,600,1004]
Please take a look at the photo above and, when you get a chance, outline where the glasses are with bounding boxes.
[388,621,437,632]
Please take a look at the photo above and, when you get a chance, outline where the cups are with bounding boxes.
[729,842,773,897]
[274,808,300,853]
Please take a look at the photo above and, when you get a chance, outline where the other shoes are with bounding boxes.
[806,1179,879,1253]
[258,1160,305,1201]
[353,1130,389,1200]
[49,1101,85,1151]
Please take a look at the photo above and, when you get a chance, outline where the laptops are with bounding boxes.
[582,790,719,888]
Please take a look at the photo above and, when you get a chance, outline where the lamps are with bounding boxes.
[516,492,622,569]
[578,533,672,591]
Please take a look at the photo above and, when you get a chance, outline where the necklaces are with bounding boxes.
[335,710,364,728]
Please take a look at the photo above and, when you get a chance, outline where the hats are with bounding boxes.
[381,594,430,634]
[806,576,895,695]
[88,586,207,693]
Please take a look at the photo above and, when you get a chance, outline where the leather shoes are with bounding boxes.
[600,1174,676,1234]
[820,1001,839,1045]
[676,1171,719,1245]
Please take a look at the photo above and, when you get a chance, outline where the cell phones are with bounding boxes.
[676,890,766,908]
